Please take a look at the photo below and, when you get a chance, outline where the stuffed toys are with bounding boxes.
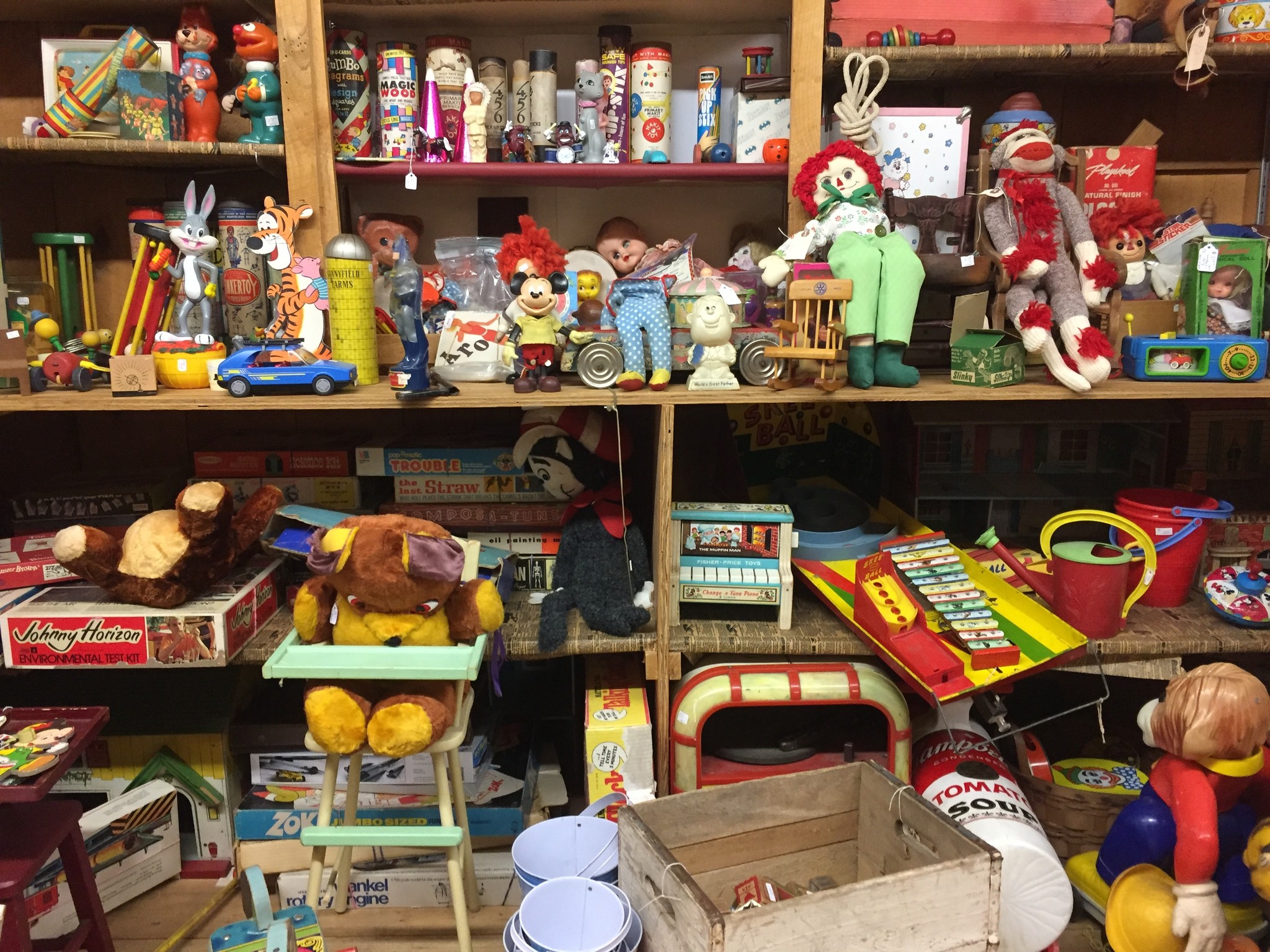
[293,513,506,757]
[513,406,656,655]
[52,480,282,611]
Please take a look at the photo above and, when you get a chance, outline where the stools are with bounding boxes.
[1,798,117,952]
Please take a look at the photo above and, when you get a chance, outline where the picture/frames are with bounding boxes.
[38,34,183,139]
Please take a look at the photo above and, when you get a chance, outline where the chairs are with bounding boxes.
[288,529,487,952]
[762,275,857,391]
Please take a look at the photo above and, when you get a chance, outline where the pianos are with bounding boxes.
[670,500,800,630]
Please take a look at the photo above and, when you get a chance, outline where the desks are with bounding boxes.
[0,703,112,805]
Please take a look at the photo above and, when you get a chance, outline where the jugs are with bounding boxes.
[975,507,1158,639]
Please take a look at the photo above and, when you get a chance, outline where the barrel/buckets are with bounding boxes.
[503,791,643,952]
[1110,487,1235,607]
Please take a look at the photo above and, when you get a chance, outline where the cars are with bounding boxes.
[212,339,359,396]
[556,275,792,388]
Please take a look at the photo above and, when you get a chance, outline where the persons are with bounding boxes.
[576,269,602,302]
[595,216,724,288]
[463,81,492,163]
[494,213,569,384]
[756,140,921,390]
[1088,196,1172,301]
[979,117,1118,394]
[221,20,285,144]
[158,616,216,664]
[601,274,678,390]
[717,220,779,324]
[1205,264,1252,336]
[684,293,737,380]
[357,211,423,282]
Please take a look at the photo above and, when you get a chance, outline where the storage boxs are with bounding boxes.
[1215,1,1270,45]
[825,0,1116,48]
[732,75,791,93]
[1180,232,1266,341]
[617,759,1002,952]
[117,66,189,142]
[948,288,1027,388]
[228,654,655,916]
[2,419,658,674]
[728,90,792,163]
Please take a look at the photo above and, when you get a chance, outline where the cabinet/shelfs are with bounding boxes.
[0,1,1269,805]
